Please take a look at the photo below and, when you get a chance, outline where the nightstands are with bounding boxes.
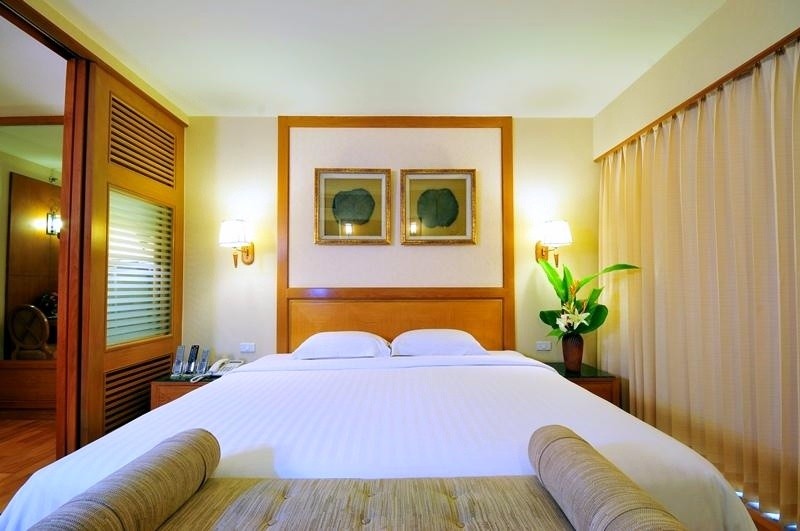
[544,362,621,408]
[150,376,219,410]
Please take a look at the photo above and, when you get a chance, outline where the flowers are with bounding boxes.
[537,257,640,344]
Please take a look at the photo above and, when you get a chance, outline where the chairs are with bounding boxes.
[8,303,55,360]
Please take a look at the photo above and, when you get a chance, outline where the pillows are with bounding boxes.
[291,331,392,358]
[388,328,491,355]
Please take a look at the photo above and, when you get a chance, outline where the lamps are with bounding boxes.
[216,217,256,268]
[535,220,573,268]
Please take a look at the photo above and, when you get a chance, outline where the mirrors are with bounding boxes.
[0,122,64,361]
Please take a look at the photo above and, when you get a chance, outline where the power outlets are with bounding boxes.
[537,342,552,351]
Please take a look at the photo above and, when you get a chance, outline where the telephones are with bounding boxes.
[205,358,244,378]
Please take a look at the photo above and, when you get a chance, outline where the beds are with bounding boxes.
[0,116,758,531]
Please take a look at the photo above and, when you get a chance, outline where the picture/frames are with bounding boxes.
[46,212,60,235]
[400,169,480,247]
[314,167,393,246]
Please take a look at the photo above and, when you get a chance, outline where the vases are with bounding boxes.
[562,331,584,373]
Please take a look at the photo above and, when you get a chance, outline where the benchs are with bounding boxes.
[26,428,692,531]
